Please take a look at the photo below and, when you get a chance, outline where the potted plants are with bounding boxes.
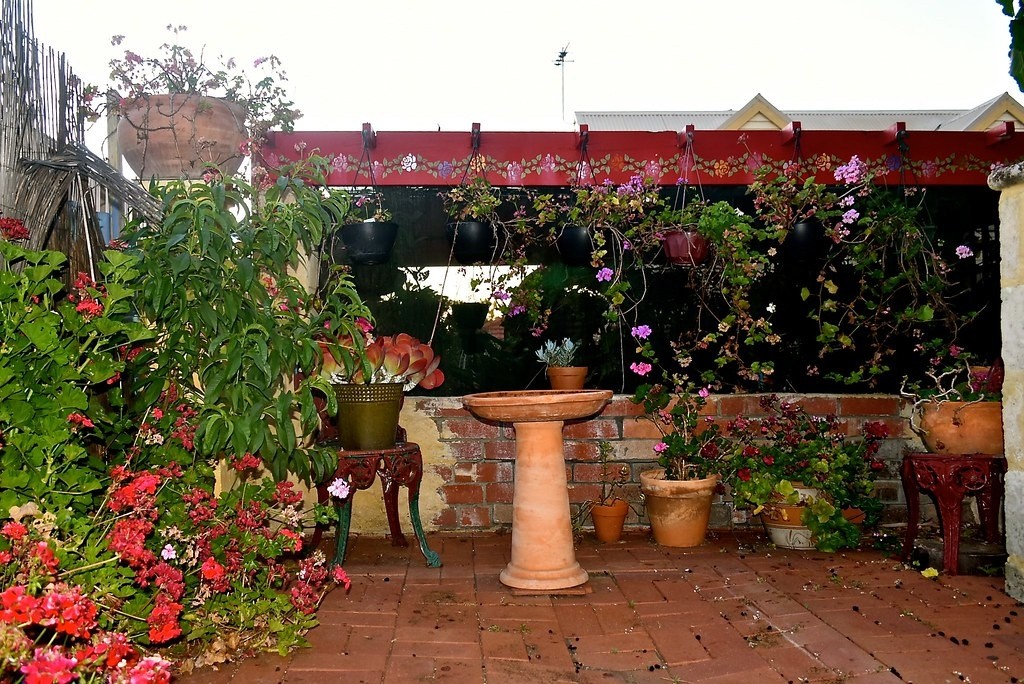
[535,337,588,391]
[569,440,645,546]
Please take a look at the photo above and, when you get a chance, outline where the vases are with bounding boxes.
[782,224,832,260]
[897,224,937,258]
[550,227,613,266]
[917,402,1005,455]
[639,469,716,548]
[339,221,399,266]
[328,383,404,450]
[760,480,835,551]
[663,231,710,265]
[116,96,249,180]
[450,303,487,329]
[444,222,510,265]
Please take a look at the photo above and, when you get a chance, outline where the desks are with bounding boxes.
[899,451,1008,574]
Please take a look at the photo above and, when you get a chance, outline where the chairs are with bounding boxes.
[292,371,443,575]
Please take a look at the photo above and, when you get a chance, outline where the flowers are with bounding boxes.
[0,24,1003,682]
